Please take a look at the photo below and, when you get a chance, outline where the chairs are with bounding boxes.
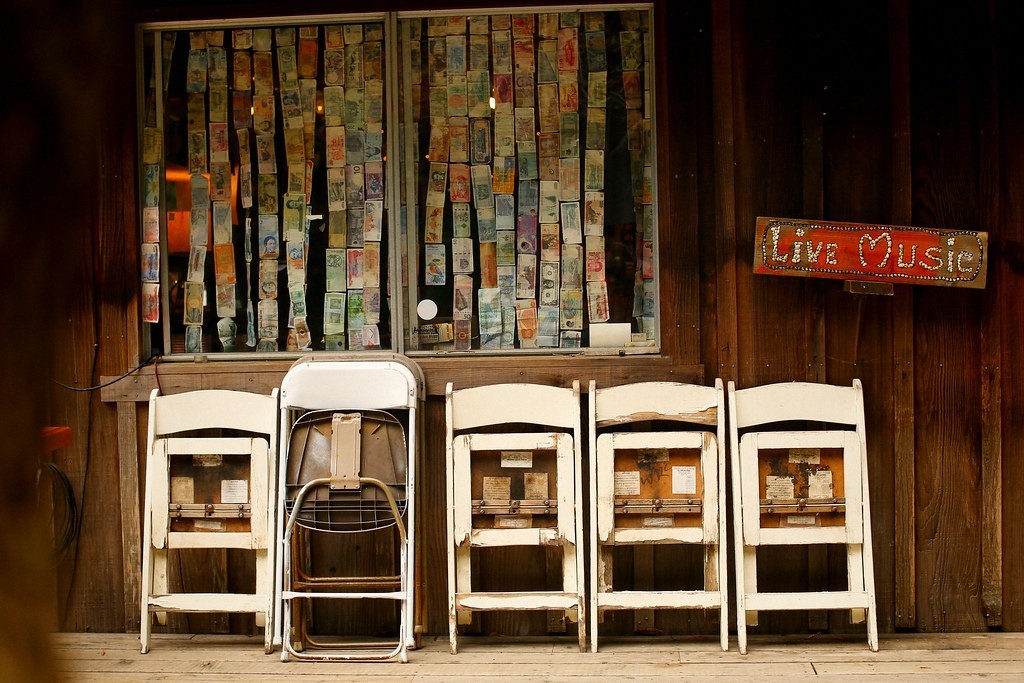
[444,378,586,654]
[728,378,878,654]
[140,388,280,656]
[589,379,730,653]
[277,351,428,664]
